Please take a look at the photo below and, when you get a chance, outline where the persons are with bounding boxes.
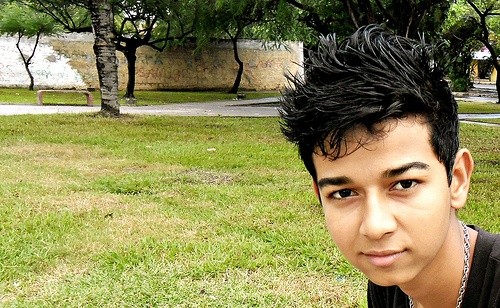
[274,23,500,308]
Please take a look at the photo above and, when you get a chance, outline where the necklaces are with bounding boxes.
[408,220,471,308]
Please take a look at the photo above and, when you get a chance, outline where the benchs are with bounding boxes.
[37,90,93,107]
